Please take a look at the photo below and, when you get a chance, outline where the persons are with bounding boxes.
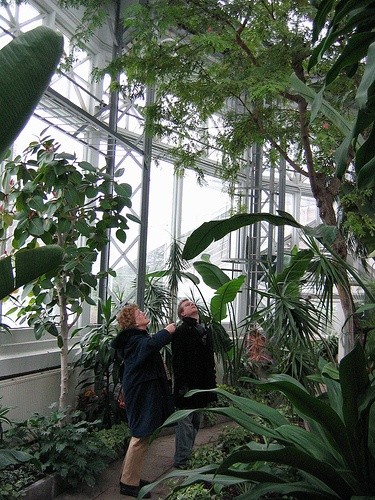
[171,297,219,471]
[110,303,177,499]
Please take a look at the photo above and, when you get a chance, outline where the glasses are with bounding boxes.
[134,311,142,319]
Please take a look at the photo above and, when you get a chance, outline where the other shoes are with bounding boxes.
[120,484,151,498]
[120,477,151,486]
[174,464,193,469]
